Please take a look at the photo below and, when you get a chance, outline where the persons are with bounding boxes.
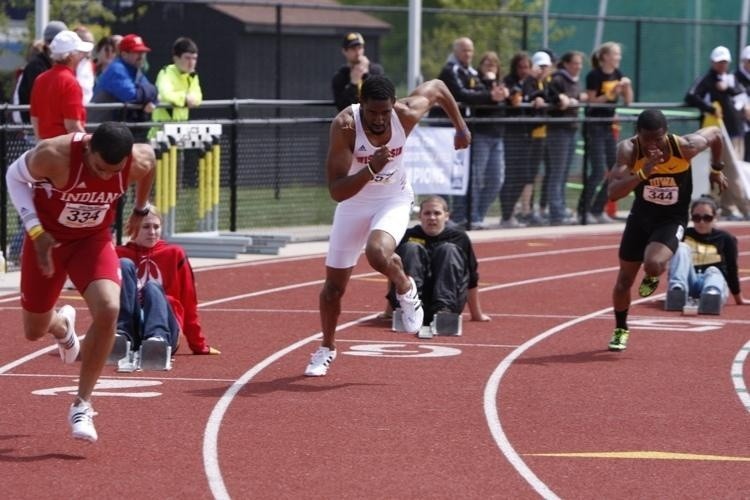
[663,194,750,315]
[303,76,473,380]
[605,107,729,351]
[431,36,634,230]
[374,194,492,340]
[684,46,750,162]
[12,21,202,263]
[332,31,385,113]
[29,27,97,291]
[4,120,158,441]
[113,201,226,374]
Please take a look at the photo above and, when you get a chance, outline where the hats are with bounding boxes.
[44,20,95,54]
[117,34,148,52]
[532,50,552,68]
[710,47,731,62]
[344,32,364,50]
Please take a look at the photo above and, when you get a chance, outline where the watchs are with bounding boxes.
[710,161,725,170]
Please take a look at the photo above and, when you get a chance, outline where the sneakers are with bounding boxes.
[501,210,613,229]
[68,399,98,442]
[608,327,629,352]
[57,305,79,363]
[638,276,659,296]
[305,347,338,377]
[394,275,425,335]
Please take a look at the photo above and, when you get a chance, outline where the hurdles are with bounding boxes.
[149,122,294,261]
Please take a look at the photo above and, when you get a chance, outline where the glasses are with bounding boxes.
[692,214,714,223]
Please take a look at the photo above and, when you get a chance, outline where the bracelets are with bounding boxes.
[639,168,648,181]
[30,230,44,240]
[384,311,393,318]
[710,167,722,174]
[636,170,644,182]
[29,225,42,237]
[132,207,150,216]
[367,161,376,177]
